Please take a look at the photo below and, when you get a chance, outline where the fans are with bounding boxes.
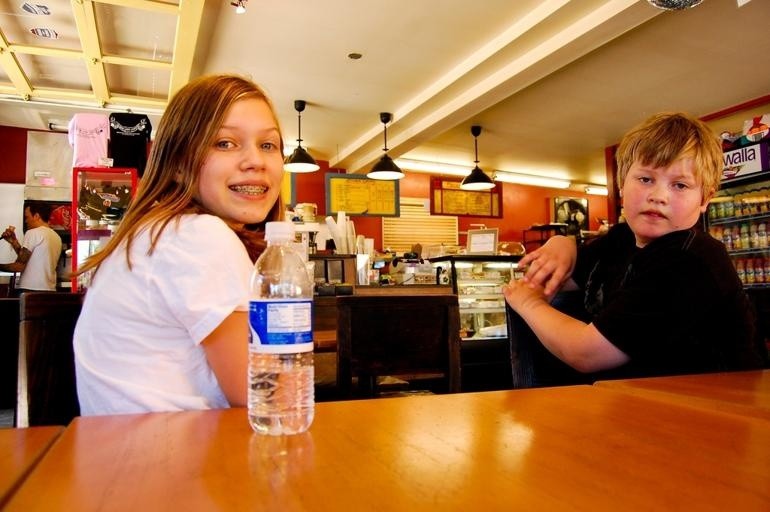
[554,196,589,235]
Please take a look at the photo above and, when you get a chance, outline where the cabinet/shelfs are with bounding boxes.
[306,221,570,394]
[70,166,139,294]
[701,139,770,291]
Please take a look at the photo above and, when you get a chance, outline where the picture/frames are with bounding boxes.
[324,172,401,218]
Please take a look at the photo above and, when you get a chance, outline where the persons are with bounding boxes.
[60,75,284,416]
[0,200,62,294]
[501,112,770,379]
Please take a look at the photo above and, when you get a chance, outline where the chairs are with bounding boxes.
[503,288,587,390]
[16,290,85,427]
[335,294,463,400]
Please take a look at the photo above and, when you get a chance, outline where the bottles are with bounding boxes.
[753,259,764,283]
[740,224,750,250]
[750,188,759,214]
[716,228,724,243]
[758,224,768,247]
[759,186,769,213]
[709,196,717,220]
[737,258,747,284]
[731,226,740,249]
[763,256,769,282]
[734,192,742,217]
[742,191,750,216]
[723,228,732,249]
[747,259,755,284]
[727,194,734,217]
[247,222,314,436]
[716,196,726,218]
[768,233,770,247]
[749,224,759,248]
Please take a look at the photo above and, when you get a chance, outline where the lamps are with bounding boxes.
[460,125,497,190]
[367,112,405,181]
[231,0,248,14]
[584,186,608,197]
[492,170,571,190]
[283,100,321,173]
